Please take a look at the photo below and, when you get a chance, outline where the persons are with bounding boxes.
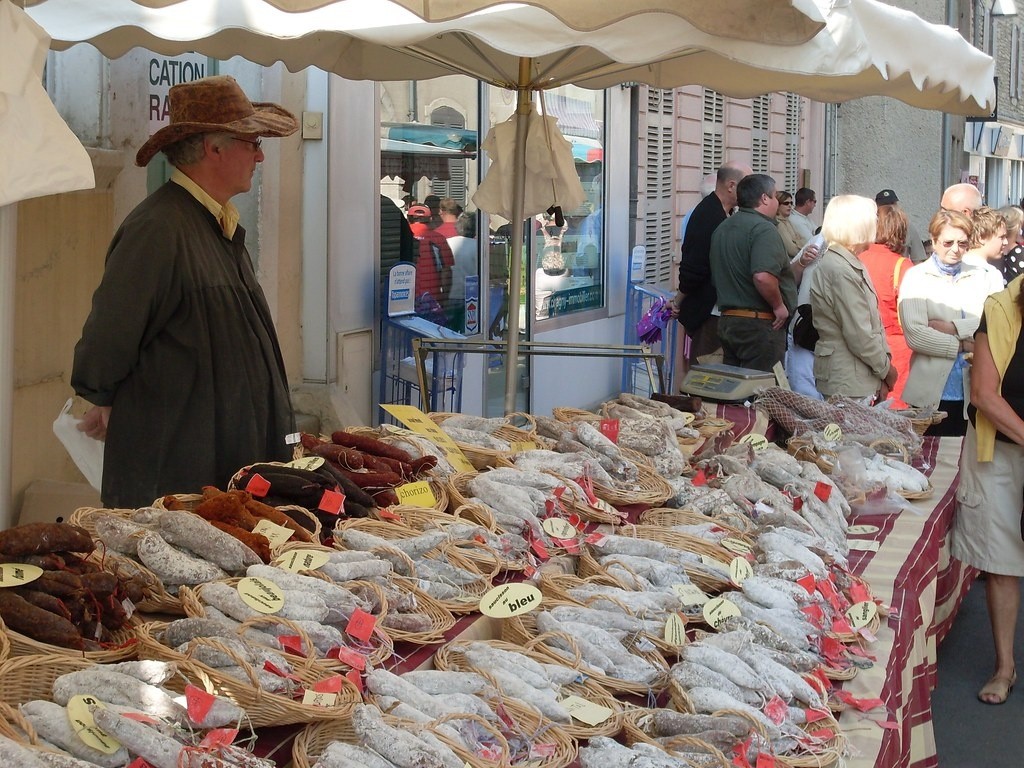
[535,202,602,320]
[71,74,298,510]
[678,161,1024,438]
[952,269,1024,706]
[381,195,479,335]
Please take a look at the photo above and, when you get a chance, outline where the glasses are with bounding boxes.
[231,136,262,152]
[937,239,968,248]
[781,201,794,206]
[810,199,817,204]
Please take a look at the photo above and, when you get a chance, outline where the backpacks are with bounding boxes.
[540,226,569,273]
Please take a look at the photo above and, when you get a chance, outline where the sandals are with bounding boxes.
[978,667,1017,704]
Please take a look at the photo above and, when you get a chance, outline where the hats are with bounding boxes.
[407,203,431,217]
[135,75,300,167]
[875,189,899,205]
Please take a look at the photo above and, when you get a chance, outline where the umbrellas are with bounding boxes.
[30,0,994,410]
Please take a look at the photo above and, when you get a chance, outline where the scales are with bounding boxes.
[679,363,777,405]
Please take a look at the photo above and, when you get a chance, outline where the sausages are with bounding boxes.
[0,383,930,768]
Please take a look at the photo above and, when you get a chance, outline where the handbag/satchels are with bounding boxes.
[636,296,672,345]
[415,291,449,327]
[893,257,907,332]
[792,303,820,351]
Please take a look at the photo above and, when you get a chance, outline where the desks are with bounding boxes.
[248,398,984,768]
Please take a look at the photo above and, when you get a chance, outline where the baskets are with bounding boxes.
[0,407,946,768]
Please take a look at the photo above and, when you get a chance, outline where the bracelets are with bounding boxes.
[962,341,965,353]
[798,257,805,266]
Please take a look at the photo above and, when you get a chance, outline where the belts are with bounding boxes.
[723,309,774,320]
